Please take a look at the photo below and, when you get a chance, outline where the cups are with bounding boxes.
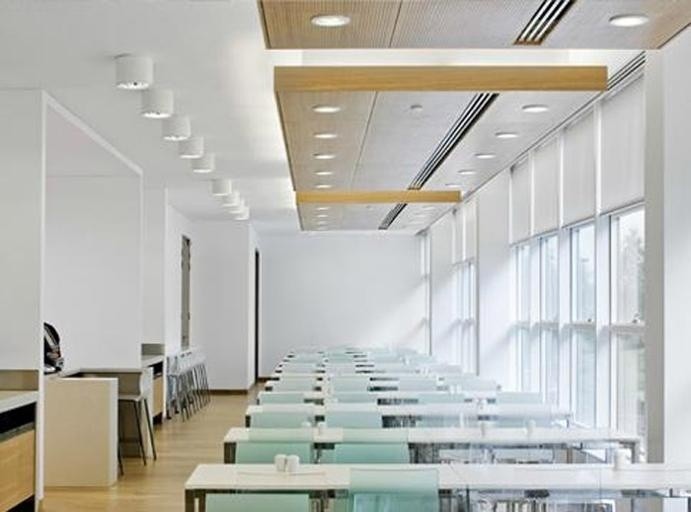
[274,454,299,472]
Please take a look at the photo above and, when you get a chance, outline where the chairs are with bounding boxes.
[182,350,691,512]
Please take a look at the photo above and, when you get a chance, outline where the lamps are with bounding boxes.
[117,57,174,119]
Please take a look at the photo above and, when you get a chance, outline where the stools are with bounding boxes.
[118,394,158,475]
